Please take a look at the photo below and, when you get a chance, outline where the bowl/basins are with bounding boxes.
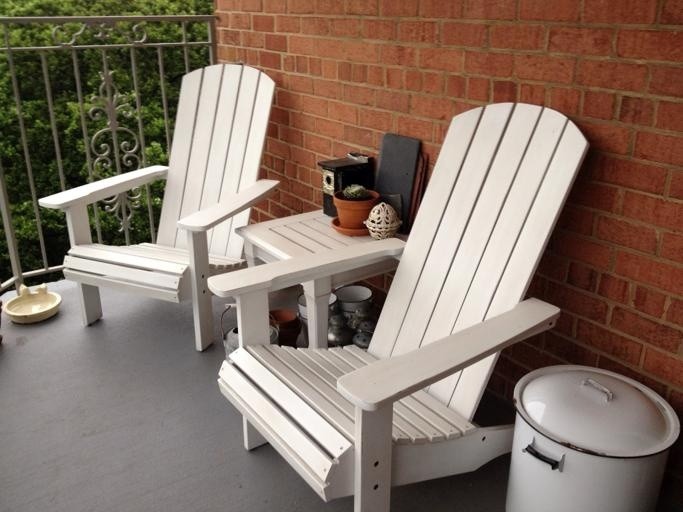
[296,284,373,321]
[1,283,62,324]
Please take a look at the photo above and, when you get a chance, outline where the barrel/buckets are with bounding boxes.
[335,285,374,326]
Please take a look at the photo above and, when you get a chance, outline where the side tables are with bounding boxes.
[233,206,406,349]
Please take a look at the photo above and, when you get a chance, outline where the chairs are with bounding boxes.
[36,65,279,350]
[203,99,593,508]
